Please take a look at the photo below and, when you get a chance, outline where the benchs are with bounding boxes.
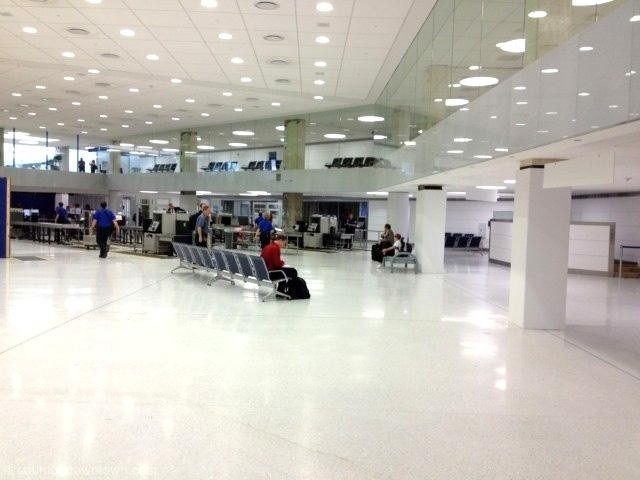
[383,241,418,274]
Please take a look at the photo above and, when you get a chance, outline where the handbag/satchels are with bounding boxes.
[275,276,310,299]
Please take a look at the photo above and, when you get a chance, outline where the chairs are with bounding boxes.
[149,164,177,172]
[171,241,293,302]
[201,160,282,171]
[325,157,375,169]
[445,233,485,256]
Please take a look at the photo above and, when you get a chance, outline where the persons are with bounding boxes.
[252,210,276,252]
[55,202,68,236]
[343,212,358,249]
[382,233,402,257]
[188,202,211,247]
[89,160,98,173]
[261,235,297,298]
[133,208,143,226]
[88,201,120,258]
[167,202,185,213]
[84,204,96,250]
[78,158,85,172]
[376,224,394,269]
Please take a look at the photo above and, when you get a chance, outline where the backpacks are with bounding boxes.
[396,238,412,257]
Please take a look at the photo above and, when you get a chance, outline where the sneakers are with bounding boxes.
[99,252,107,258]
[377,264,382,269]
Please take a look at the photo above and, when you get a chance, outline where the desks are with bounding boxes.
[323,232,355,252]
